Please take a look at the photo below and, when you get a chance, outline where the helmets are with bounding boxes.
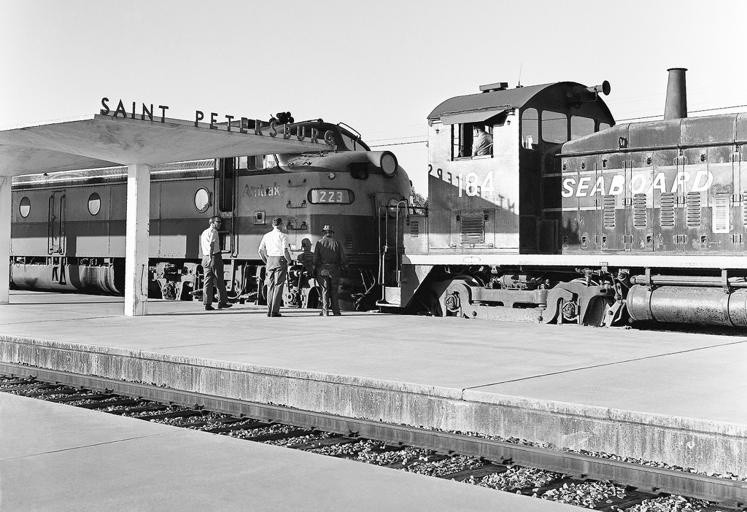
[322,224,334,233]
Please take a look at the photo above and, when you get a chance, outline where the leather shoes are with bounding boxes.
[205,306,215,310]
[218,303,232,308]
[268,313,281,317]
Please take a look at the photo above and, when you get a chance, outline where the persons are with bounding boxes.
[310,225,346,315]
[257,218,293,317]
[471,123,492,157]
[199,216,233,311]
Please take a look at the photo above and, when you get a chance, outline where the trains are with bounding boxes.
[372,66,746,331]
[9,145,417,314]
